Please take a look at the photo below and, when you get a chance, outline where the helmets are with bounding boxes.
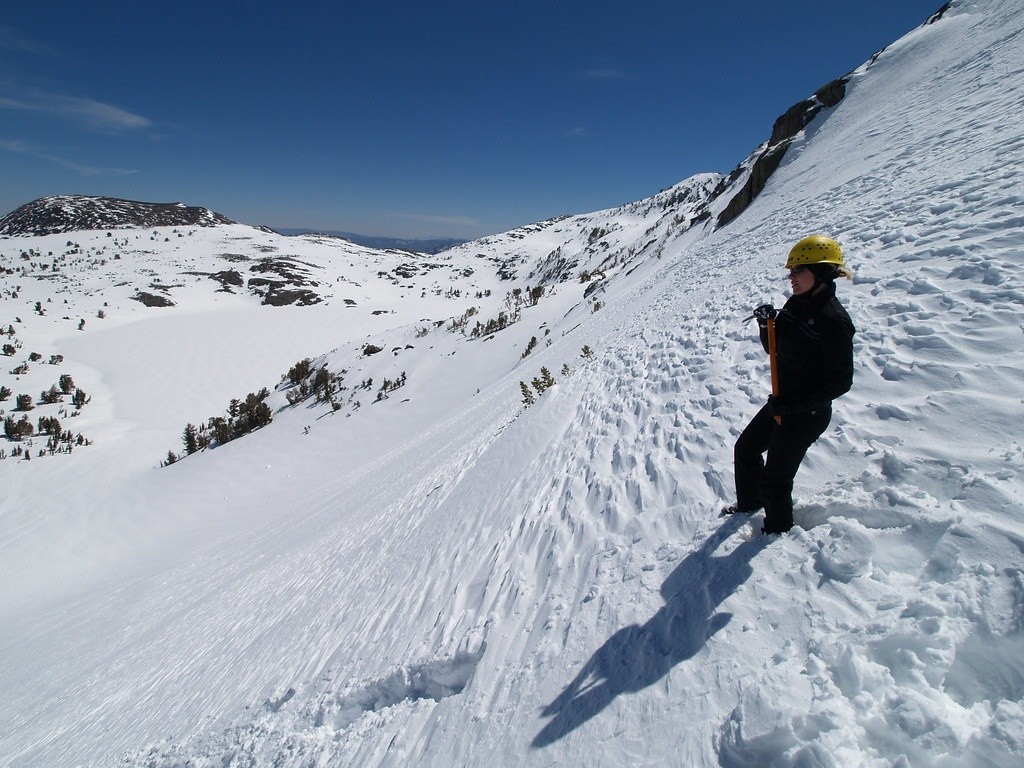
[785,234,844,269]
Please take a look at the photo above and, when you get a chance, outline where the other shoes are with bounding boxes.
[722,502,761,513]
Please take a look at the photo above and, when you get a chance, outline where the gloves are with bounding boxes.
[755,305,776,325]
[769,391,789,416]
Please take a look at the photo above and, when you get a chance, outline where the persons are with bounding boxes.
[722,235,854,535]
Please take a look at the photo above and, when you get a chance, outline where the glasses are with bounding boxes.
[790,266,810,274]
